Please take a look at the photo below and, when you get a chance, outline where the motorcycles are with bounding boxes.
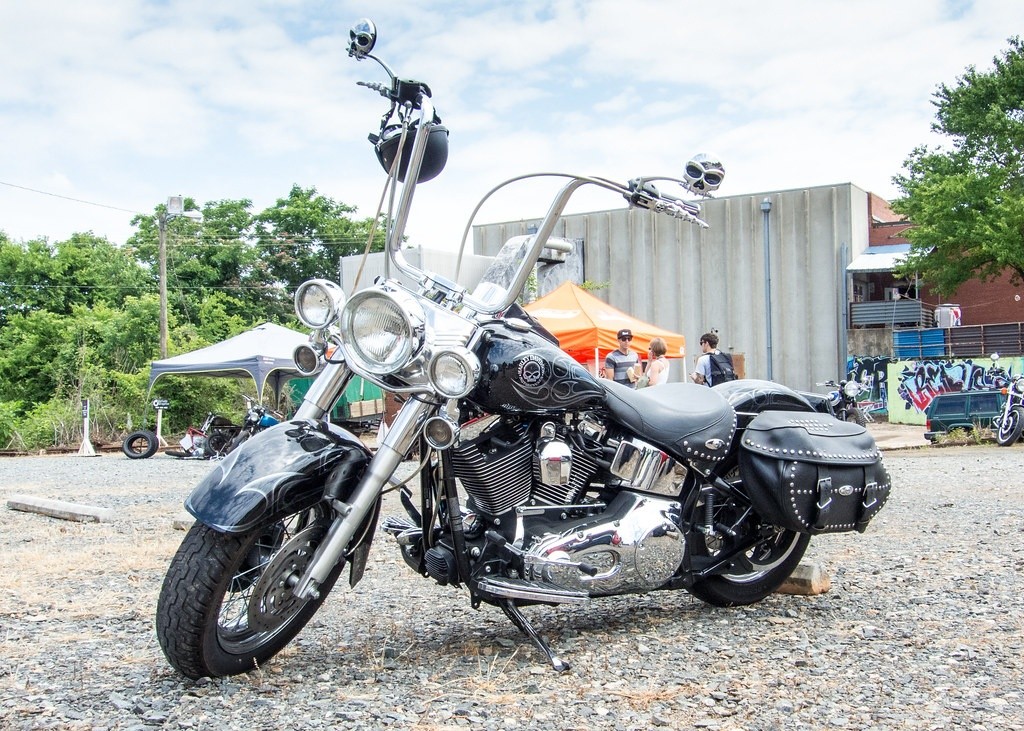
[993,368,1024,448]
[814,362,871,428]
[156,18,866,680]
[206,392,285,458]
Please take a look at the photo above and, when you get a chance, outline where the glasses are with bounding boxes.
[619,337,632,341]
[700,342,706,345]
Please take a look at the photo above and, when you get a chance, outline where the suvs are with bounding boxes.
[924,387,1024,444]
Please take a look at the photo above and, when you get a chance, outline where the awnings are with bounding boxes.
[845,244,941,303]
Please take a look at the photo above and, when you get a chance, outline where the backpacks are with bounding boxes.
[703,349,736,387]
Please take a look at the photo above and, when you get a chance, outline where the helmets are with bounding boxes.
[374,125,449,183]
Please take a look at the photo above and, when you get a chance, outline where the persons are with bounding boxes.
[604,329,643,389]
[689,332,722,388]
[646,337,669,386]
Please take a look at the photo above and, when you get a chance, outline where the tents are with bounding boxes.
[139,322,336,454]
[522,282,688,384]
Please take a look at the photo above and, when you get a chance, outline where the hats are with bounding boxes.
[618,330,633,340]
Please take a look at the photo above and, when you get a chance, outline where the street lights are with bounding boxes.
[759,201,772,381]
[159,195,205,360]
